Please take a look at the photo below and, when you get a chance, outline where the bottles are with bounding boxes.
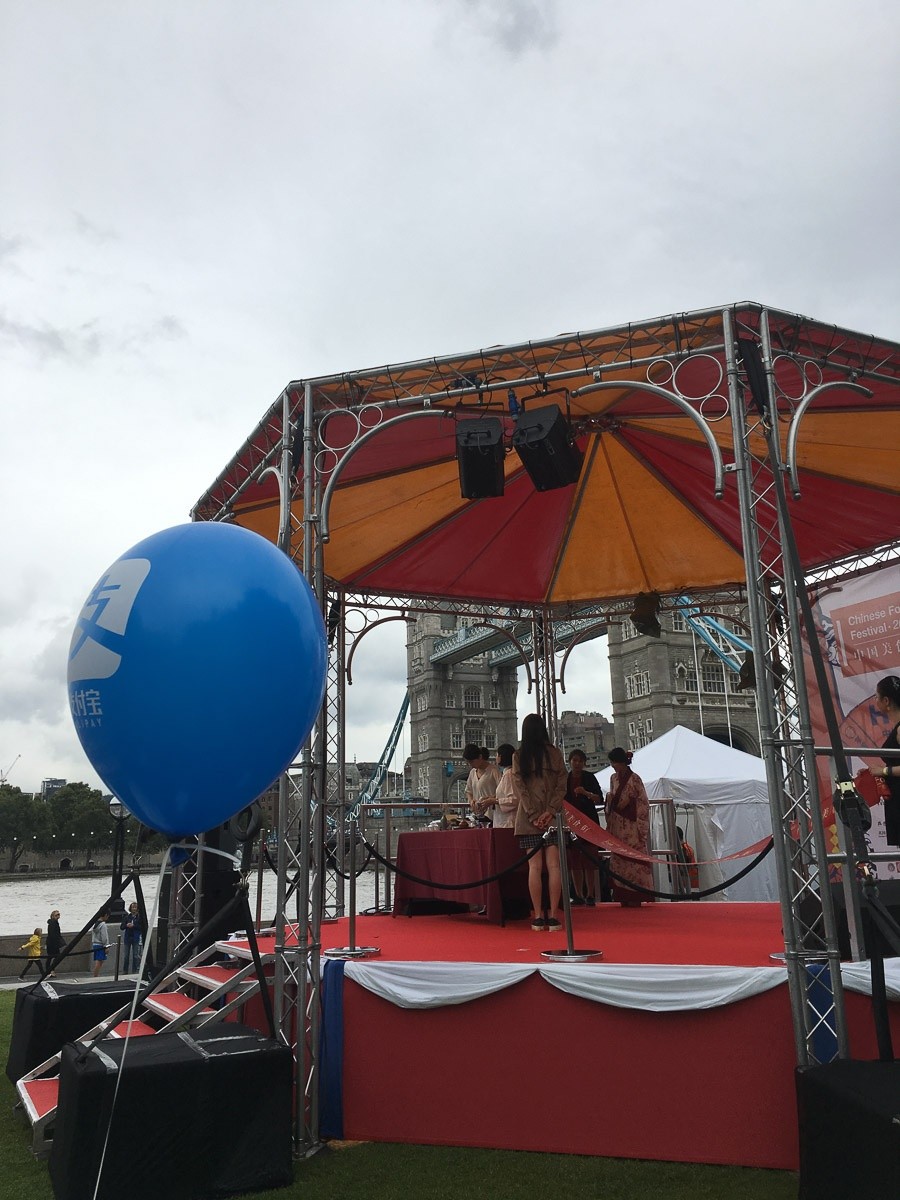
[440,815,447,831]
[458,818,468,828]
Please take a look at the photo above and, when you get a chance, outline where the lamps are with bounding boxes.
[513,387,585,492]
[455,418,506,500]
[736,650,789,690]
[629,592,661,639]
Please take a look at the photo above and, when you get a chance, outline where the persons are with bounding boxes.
[512,714,568,931]
[92,908,112,977]
[462,743,502,819]
[46,910,66,978]
[479,747,490,760]
[869,675,900,848]
[563,749,604,907]
[120,902,142,975]
[479,744,516,828]
[17,928,51,982]
[676,826,699,888]
[604,747,655,908]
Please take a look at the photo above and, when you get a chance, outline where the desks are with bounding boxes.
[394,827,564,928]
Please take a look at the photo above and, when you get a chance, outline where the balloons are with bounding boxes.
[67,521,329,869]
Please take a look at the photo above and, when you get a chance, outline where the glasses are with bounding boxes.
[55,914,59,916]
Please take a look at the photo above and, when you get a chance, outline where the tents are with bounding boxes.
[593,724,820,902]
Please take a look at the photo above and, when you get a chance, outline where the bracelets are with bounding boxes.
[888,766,892,776]
[496,800,499,804]
[883,767,888,775]
[588,793,593,799]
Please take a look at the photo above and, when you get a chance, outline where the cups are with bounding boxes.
[472,820,491,828]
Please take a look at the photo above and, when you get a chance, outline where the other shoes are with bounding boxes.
[44,974,51,980]
[547,918,563,933]
[586,896,595,906]
[51,974,57,977]
[570,896,587,905]
[531,917,545,932]
[18,978,26,982]
